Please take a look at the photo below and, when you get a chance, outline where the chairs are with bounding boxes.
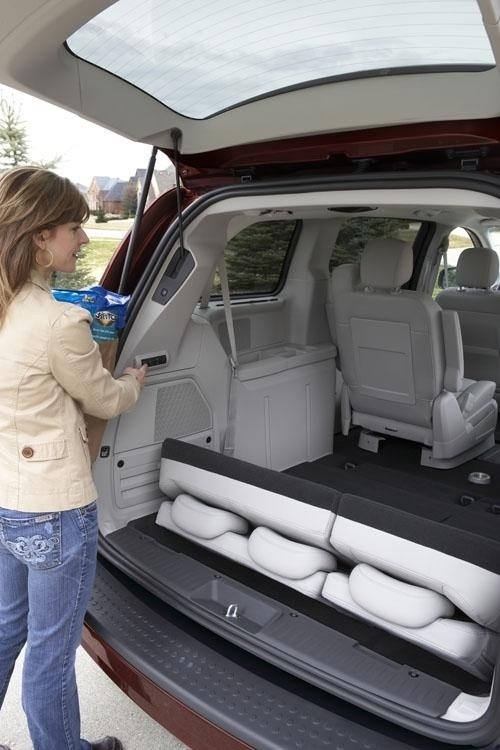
[325,238,500,469]
[123,437,500,699]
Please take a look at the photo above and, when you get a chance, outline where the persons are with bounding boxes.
[0,166,149,750]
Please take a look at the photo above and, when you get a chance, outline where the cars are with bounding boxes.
[1,1,499,749]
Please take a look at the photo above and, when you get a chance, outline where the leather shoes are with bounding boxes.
[0,734,124,750]
